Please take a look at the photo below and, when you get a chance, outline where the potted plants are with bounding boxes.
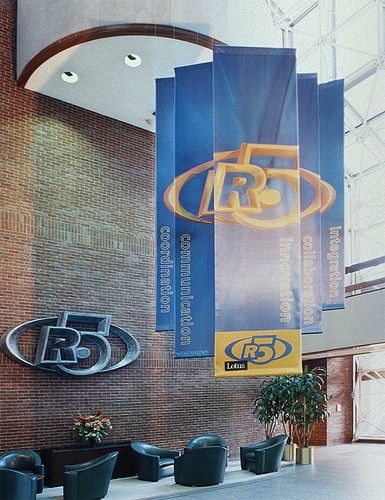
[254,366,330,465]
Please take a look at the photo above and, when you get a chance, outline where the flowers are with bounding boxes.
[73,408,112,447]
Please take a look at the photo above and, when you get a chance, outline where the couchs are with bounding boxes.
[130,441,181,482]
[63,451,119,499]
[240,435,288,475]
[174,435,227,488]
[0,450,45,500]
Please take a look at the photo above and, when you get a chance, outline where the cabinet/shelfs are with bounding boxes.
[40,441,140,488]
